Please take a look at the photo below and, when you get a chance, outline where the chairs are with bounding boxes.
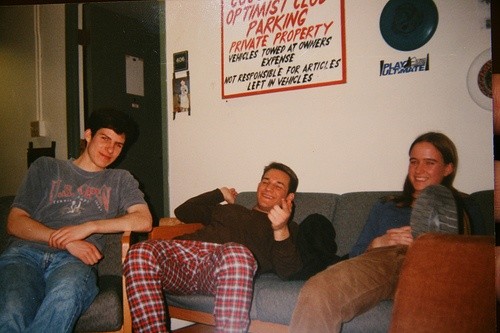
[0,195,132,332]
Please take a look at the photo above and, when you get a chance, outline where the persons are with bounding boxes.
[0,110,154,333]
[289,131,471,333]
[121,161,308,333]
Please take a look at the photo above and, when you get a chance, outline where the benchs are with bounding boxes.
[147,191,497,333]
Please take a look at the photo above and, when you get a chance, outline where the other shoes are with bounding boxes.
[410,185,464,240]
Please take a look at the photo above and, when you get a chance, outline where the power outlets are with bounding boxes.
[30,120,46,139]
